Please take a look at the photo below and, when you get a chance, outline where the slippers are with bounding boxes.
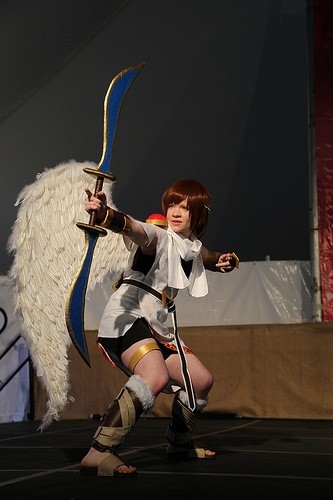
[80,450,137,478]
[167,440,216,459]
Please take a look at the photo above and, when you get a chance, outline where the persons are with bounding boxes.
[76,177,240,479]
